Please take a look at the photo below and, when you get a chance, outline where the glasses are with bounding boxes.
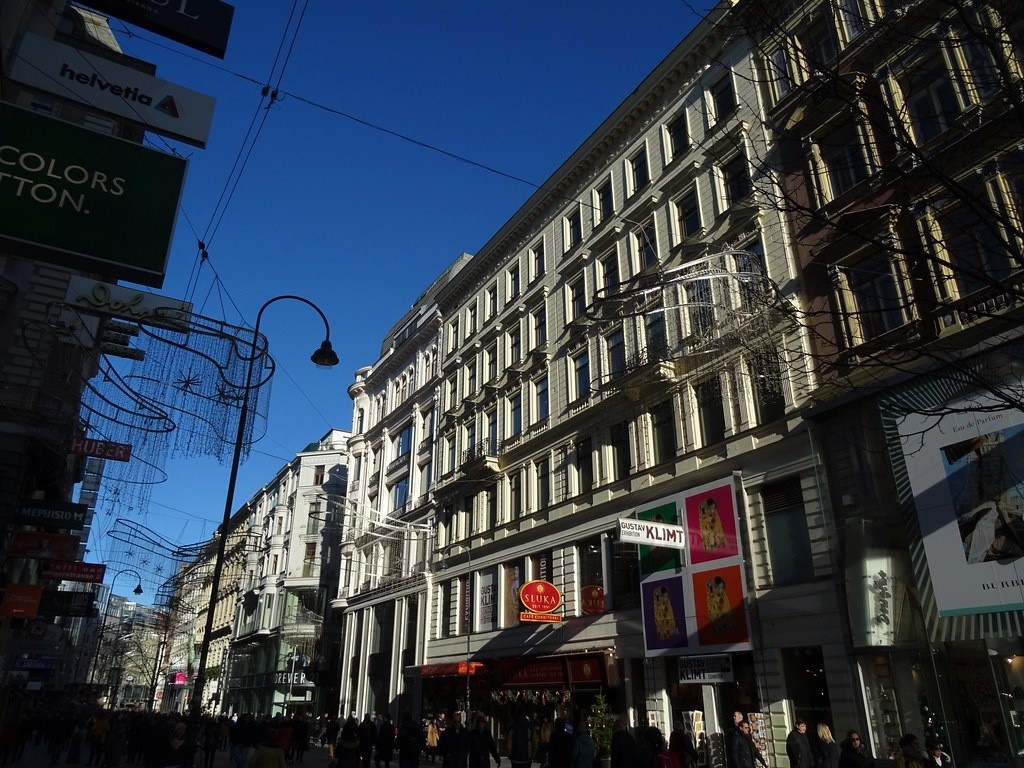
[935,748,941,751]
[745,726,749,729]
[454,721,461,724]
[852,738,859,741]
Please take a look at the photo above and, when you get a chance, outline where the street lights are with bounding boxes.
[88,569,145,700]
[438,545,470,716]
[176,294,341,768]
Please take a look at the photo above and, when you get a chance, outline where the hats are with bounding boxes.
[175,722,186,730]
[477,716,485,724]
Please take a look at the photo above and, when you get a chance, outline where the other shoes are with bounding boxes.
[295,757,299,760]
[426,755,429,761]
[300,757,303,761]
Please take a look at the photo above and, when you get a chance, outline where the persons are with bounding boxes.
[838,730,897,768]
[0,674,313,768]
[925,738,950,768]
[896,734,929,768]
[610,718,695,768]
[318,711,423,768]
[786,719,838,768]
[423,709,594,768]
[730,710,768,768]
